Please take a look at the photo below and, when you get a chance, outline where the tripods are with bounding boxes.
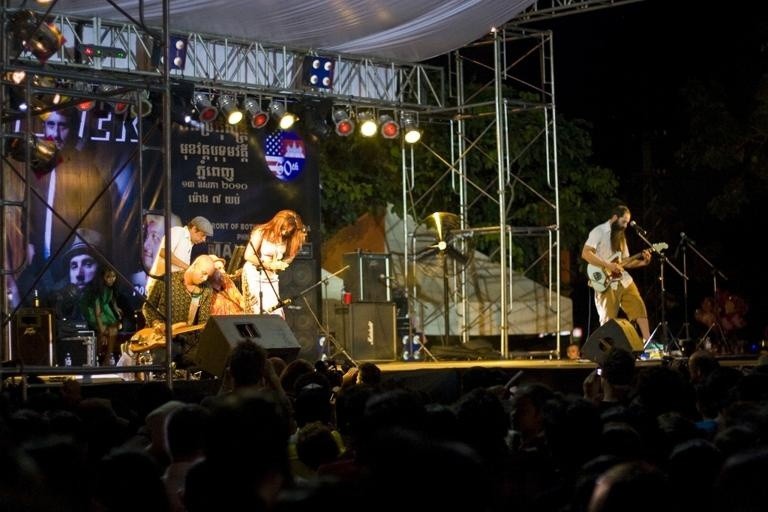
[641,237,734,358]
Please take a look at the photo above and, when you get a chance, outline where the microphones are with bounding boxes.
[268,297,293,313]
[630,221,646,235]
[681,231,696,247]
[379,273,395,280]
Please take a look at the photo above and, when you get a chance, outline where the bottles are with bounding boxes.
[135,350,155,383]
[33,288,40,307]
[64,352,72,365]
[105,352,115,367]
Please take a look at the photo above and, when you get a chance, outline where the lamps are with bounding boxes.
[3,6,161,180]
[323,91,425,147]
[180,76,303,132]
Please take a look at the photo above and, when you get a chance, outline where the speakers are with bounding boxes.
[342,252,392,303]
[15,307,52,368]
[335,301,397,363]
[278,258,321,359]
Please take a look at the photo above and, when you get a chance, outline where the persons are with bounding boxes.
[145,215,214,301]
[28,104,114,268]
[130,210,183,296]
[208,253,247,316]
[79,265,123,366]
[62,228,107,295]
[144,254,215,378]
[581,205,651,343]
[240,209,307,320]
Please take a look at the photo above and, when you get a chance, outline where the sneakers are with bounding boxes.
[644,341,664,354]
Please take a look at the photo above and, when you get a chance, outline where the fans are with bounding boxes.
[410,210,478,361]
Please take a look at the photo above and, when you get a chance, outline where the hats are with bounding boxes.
[208,254,227,266]
[188,216,214,238]
[62,228,115,271]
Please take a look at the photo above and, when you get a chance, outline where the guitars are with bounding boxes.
[129,322,207,353]
[586,241,669,293]
